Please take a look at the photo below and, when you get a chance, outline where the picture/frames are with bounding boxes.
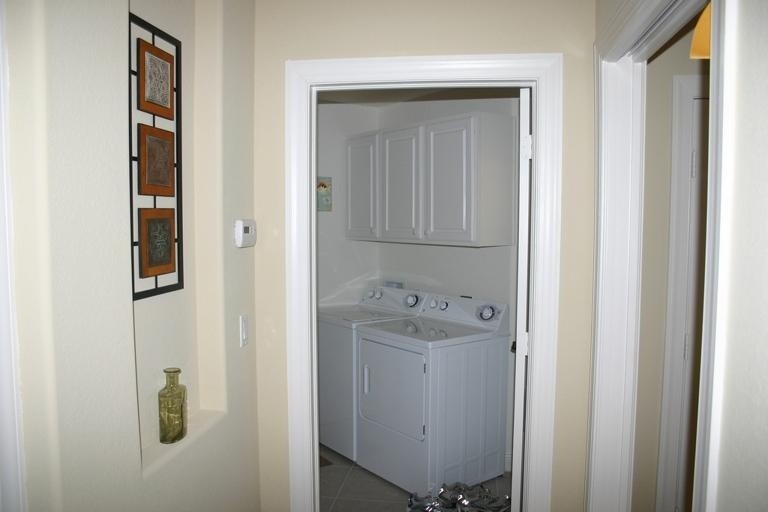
[137,123,175,197]
[136,37,175,122]
[137,208,176,279]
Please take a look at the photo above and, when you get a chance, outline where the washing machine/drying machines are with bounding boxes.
[318,286,428,464]
[356,294,510,498]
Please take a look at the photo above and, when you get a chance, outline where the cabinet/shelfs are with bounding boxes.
[378,111,514,248]
[344,130,378,241]
